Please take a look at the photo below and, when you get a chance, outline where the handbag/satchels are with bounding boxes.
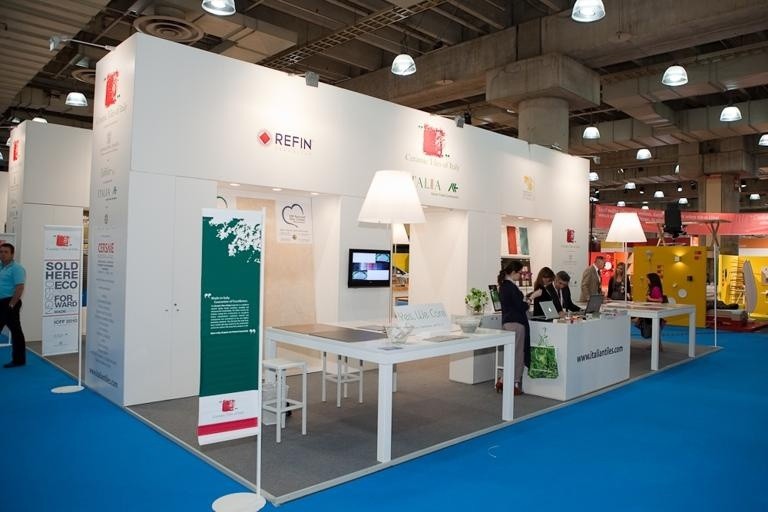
[640,318,662,339]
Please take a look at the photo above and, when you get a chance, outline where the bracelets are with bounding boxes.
[528,297,532,302]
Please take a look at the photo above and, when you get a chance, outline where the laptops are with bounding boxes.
[531,300,560,322]
[584,294,604,316]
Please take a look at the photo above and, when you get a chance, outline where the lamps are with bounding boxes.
[390,31,417,77]
[28,76,88,124]
[605,212,647,304]
[571,0,743,140]
[357,167,427,333]
[201,0,237,18]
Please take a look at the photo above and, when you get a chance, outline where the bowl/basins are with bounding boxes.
[457,319,480,333]
[383,326,414,345]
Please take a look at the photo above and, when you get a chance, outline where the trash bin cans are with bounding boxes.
[262,382,289,425]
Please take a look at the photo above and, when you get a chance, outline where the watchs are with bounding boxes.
[9,304,14,307]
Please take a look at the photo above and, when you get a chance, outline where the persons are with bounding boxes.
[635,272,669,352]
[533,266,567,318]
[579,255,606,303]
[607,261,634,301]
[552,270,585,315]
[0,243,27,367]
[496,260,543,394]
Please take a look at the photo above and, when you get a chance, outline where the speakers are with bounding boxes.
[664,207,681,233]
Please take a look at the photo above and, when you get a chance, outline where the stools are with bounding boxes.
[260,358,308,443]
[322,351,364,408]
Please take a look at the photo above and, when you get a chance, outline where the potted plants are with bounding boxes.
[464,287,490,315]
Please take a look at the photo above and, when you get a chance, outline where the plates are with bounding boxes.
[668,298,676,304]
[679,289,688,298]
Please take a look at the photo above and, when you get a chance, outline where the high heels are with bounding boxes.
[497,382,503,392]
[515,386,522,395]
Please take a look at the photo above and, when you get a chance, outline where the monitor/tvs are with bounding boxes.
[348,249,391,287]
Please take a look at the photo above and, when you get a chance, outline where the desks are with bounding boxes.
[263,322,518,462]
[576,299,696,371]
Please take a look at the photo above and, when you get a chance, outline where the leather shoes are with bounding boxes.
[4,360,26,367]
